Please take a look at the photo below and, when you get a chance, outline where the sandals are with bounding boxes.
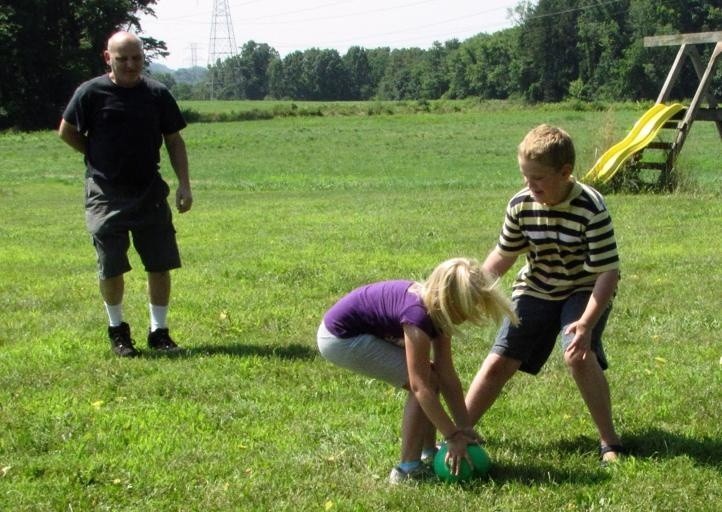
[599,440,623,468]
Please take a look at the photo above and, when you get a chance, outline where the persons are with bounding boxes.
[461,123,629,465]
[58,31,193,355]
[318,257,524,486]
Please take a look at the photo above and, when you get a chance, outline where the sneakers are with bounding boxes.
[147,328,176,350]
[109,322,138,356]
[388,448,437,484]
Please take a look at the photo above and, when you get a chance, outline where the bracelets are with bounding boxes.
[444,432,464,439]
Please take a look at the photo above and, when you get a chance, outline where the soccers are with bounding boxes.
[433,442,489,483]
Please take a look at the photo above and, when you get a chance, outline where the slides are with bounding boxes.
[579,102,685,184]
